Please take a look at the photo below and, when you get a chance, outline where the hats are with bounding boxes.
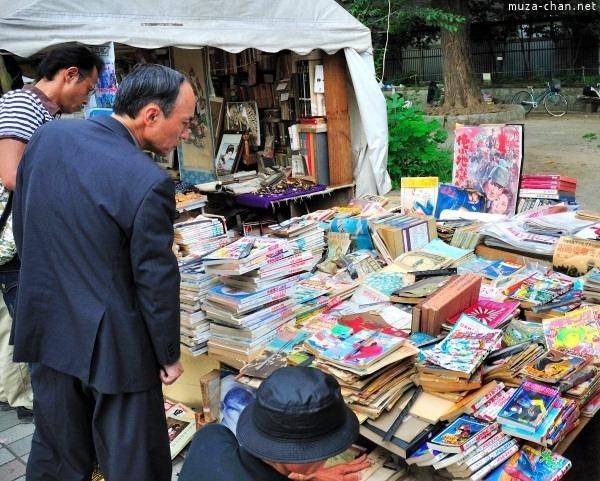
[237,366,359,464]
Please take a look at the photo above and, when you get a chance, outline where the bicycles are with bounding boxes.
[510,77,571,121]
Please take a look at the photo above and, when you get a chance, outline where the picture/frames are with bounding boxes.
[214,130,247,175]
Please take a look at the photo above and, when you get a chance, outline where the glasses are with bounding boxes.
[78,72,96,96]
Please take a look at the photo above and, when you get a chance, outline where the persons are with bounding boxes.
[12,64,197,481]
[0,44,103,421]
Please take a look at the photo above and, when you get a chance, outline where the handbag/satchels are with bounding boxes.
[0,183,18,266]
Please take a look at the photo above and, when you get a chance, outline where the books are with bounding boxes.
[204,49,329,186]
